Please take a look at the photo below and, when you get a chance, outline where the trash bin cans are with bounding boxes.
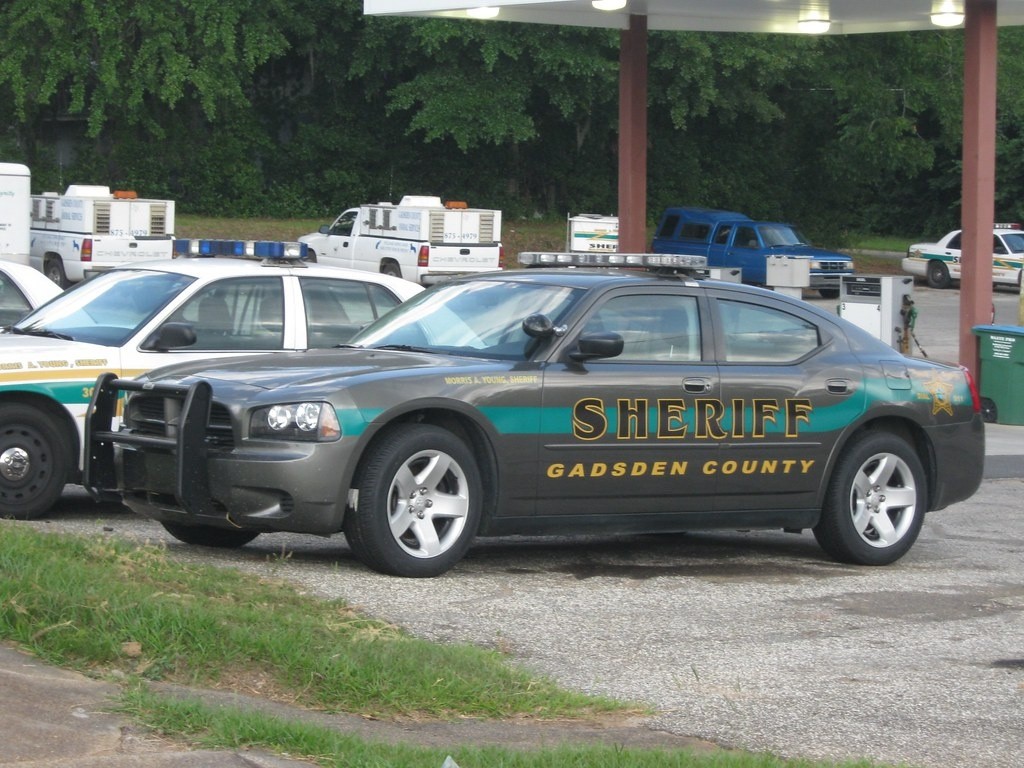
[970,323,1024,428]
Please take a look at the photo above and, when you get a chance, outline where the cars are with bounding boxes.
[0,261,97,331]
[82,248,986,580]
[0,255,492,522]
[903,222,1024,290]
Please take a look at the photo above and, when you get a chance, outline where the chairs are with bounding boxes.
[654,306,689,362]
[197,295,283,348]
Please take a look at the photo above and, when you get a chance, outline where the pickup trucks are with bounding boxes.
[293,195,504,288]
[31,184,178,289]
[649,206,856,299]
